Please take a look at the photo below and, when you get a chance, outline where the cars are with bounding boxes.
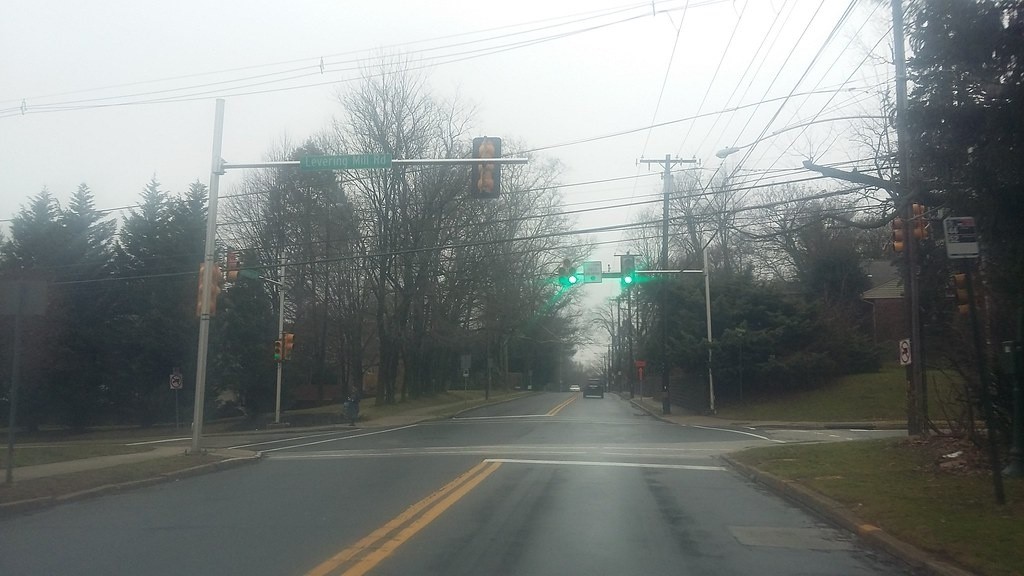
[583,379,604,397]
[569,384,579,392]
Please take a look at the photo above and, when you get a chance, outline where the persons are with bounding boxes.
[343,384,360,426]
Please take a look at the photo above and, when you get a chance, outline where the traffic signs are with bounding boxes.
[944,213,981,259]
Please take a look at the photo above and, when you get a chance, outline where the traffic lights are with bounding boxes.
[283,332,294,360]
[951,269,970,317]
[274,339,282,365]
[621,256,636,287]
[891,215,905,251]
[558,273,577,285]
